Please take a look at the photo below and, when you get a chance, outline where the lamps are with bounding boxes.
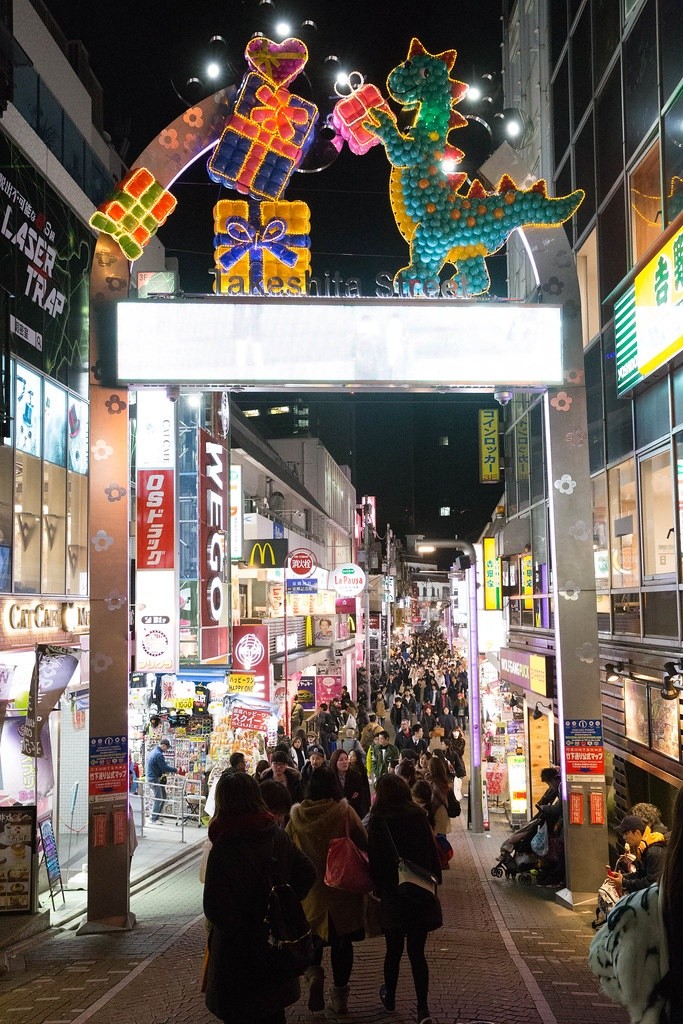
[509,691,526,707]
[245,497,270,508]
[660,673,683,700]
[617,659,630,672]
[605,661,624,683]
[534,700,551,719]
[275,509,305,519]
[663,658,683,676]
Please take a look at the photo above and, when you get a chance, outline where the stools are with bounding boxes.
[182,791,207,827]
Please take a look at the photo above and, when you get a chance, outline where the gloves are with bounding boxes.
[178,768,185,776]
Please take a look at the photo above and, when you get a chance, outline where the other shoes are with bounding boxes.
[150,819,163,824]
[378,983,396,1013]
[417,1009,432,1024]
[536,879,563,889]
[496,855,510,864]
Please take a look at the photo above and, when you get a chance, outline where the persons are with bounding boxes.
[146,738,180,825]
[222,626,468,875]
[364,775,443,1024]
[129,756,138,880]
[314,619,333,646]
[607,781,683,1024]
[283,765,369,1013]
[535,765,565,888]
[203,771,317,1024]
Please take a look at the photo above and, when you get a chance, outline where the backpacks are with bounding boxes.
[435,777,461,818]
[363,726,378,752]
[322,712,336,732]
[229,826,314,972]
[591,878,620,931]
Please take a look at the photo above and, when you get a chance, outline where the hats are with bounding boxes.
[307,730,318,739]
[612,815,644,834]
[394,758,415,778]
[161,739,172,750]
[308,747,324,758]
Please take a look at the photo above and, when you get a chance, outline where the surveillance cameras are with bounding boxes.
[494,392,513,406]
[167,387,180,402]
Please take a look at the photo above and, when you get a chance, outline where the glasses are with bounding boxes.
[272,762,286,769]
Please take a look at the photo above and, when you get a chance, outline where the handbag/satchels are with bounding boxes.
[324,807,373,893]
[531,820,549,857]
[397,861,438,912]
[158,775,169,786]
[434,833,453,865]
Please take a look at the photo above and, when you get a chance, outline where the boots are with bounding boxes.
[326,985,350,1015]
[304,965,325,1011]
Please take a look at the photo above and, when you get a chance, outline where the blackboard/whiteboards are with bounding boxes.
[38,814,61,884]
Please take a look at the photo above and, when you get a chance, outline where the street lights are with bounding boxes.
[414,540,486,833]
[436,599,453,651]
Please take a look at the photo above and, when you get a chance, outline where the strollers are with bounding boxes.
[490,803,547,886]
